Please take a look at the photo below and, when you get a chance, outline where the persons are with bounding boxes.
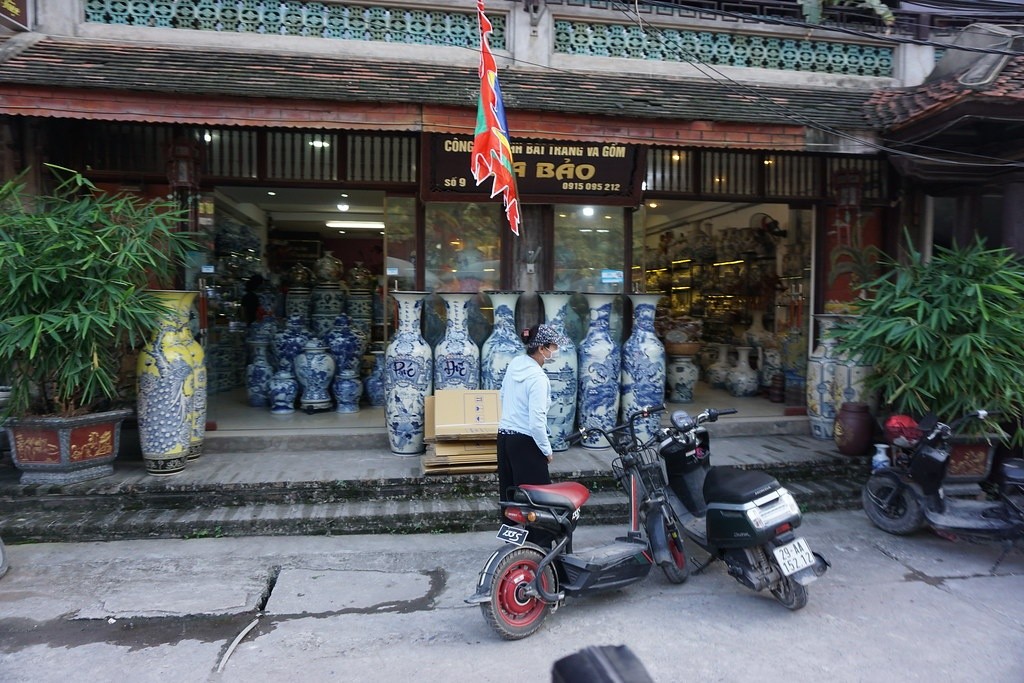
[496,324,569,528]
[243,275,265,327]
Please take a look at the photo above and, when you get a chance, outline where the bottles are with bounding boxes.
[137,247,890,476]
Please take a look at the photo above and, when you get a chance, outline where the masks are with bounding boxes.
[541,346,559,364]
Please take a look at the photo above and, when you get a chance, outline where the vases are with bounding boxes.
[213,245,785,462]
[134,290,208,477]
[806,312,883,455]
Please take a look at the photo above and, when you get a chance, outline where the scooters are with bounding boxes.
[861,408,1024,573]
[461,402,691,642]
[644,407,833,610]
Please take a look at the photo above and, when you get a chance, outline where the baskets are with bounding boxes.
[612,448,659,495]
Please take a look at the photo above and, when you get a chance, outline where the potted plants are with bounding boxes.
[0,159,213,486]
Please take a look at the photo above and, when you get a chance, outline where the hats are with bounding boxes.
[532,324,568,346]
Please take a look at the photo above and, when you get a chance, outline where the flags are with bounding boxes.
[471,1,520,236]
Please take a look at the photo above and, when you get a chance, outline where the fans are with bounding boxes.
[747,212,786,249]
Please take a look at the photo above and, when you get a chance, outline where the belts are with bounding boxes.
[498,429,523,434]
